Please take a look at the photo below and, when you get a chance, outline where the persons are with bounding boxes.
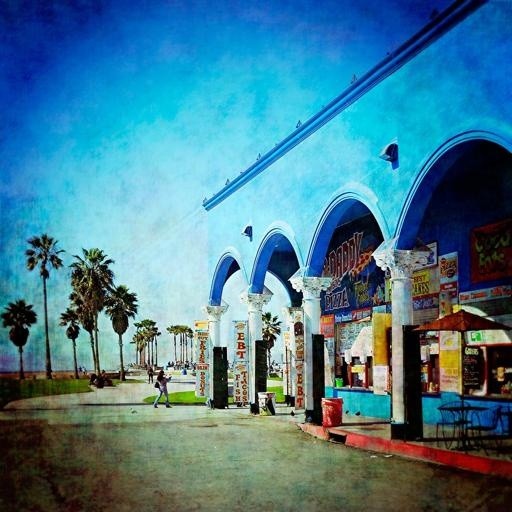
[147,366,154,384]
[153,370,172,408]
[80,366,114,388]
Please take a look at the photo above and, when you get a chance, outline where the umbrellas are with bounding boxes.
[412,310,511,398]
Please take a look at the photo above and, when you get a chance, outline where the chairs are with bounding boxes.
[437,399,512,458]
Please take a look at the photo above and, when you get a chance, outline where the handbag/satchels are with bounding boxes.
[155,382,160,388]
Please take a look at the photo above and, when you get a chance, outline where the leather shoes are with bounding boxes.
[155,406,158,408]
[166,406,172,408]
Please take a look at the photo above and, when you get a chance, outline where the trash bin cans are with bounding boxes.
[258,392,275,415]
[228,385,234,396]
[321,398,343,428]
